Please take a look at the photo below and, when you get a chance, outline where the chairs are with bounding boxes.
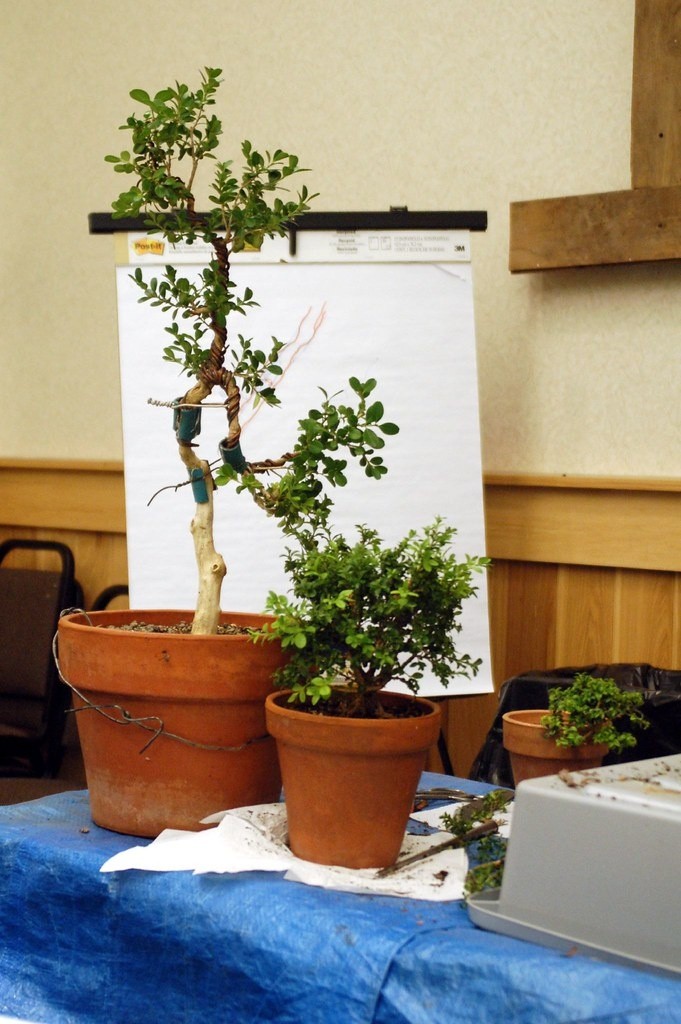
[0,540,138,793]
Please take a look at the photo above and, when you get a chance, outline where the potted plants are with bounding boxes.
[56,62,399,837]
[250,514,497,865]
[499,671,653,793]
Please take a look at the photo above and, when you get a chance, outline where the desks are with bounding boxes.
[470,664,680,787]
[0,761,679,1023]
